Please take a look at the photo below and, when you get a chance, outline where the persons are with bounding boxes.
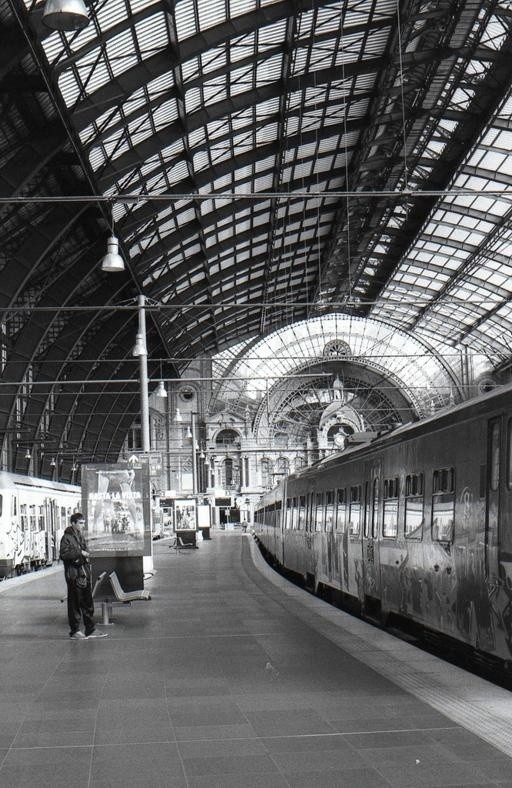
[91,469,143,540]
[59,513,109,640]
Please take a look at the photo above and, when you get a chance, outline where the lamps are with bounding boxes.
[24,449,77,472]
[41,0,89,32]
[132,334,148,357]
[156,381,168,398]
[101,236,126,273]
[173,408,182,422]
[184,426,216,476]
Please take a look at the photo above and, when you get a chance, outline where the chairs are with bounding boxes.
[178,536,194,549]
[167,539,176,547]
[61,570,158,626]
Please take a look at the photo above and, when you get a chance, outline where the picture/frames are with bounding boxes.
[196,504,212,529]
[79,462,151,558]
[172,497,198,533]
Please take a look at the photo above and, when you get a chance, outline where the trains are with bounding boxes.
[250,382,512,675]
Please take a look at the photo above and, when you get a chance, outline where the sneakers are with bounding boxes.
[88,629,108,638]
[70,630,88,640]
